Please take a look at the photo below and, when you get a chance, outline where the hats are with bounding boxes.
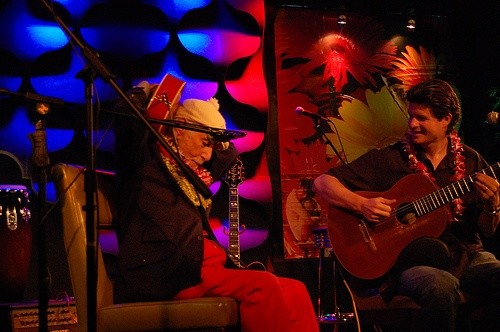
[174,97,226,134]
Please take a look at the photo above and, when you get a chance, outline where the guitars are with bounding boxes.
[223,158,267,272]
[327,164,496,282]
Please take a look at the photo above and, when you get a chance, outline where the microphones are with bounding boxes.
[295,107,330,121]
[488,111,500,124]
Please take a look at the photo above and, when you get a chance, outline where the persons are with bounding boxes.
[108,79,320,332]
[310,79,500,332]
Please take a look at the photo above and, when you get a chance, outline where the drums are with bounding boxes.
[1,184,34,303]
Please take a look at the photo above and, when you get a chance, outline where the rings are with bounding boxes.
[484,186,489,192]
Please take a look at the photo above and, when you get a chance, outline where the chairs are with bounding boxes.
[328,248,465,332]
[53,164,239,332]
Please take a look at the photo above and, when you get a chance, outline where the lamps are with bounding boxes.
[406,12,416,29]
[337,8,347,25]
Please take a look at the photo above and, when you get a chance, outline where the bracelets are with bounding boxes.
[484,208,500,217]
